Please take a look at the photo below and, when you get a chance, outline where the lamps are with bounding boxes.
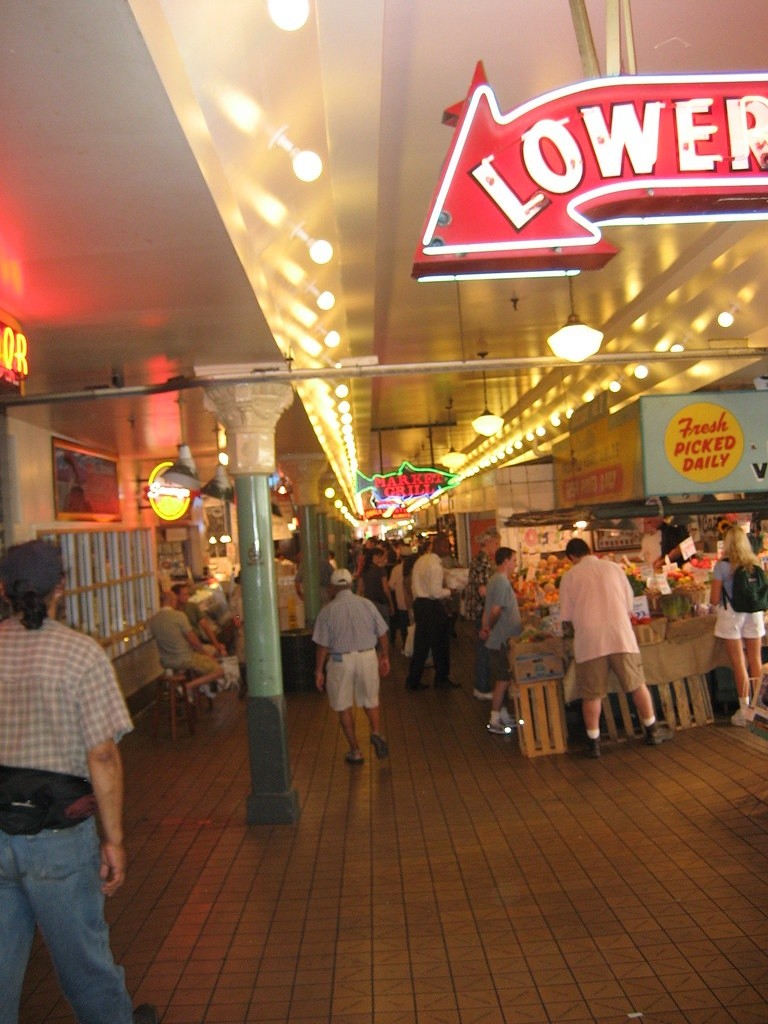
[200,417,235,505]
[441,407,466,469]
[470,353,505,437]
[547,276,604,363]
[162,389,200,492]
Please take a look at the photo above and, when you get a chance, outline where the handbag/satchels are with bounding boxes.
[0,766,97,835]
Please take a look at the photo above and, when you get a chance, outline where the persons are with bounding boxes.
[640,516,691,570]
[230,571,250,700]
[746,521,764,553]
[709,526,765,726]
[468,530,503,698]
[312,569,390,765]
[479,546,522,735]
[560,538,674,758]
[405,537,462,691]
[274,533,461,655]
[0,541,160,1024]
[150,584,226,704]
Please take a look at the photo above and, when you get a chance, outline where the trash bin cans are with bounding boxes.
[280,632,317,696]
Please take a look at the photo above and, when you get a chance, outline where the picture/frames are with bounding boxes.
[591,530,641,552]
[50,436,123,524]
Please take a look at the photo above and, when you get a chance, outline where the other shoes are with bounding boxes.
[644,723,674,744]
[183,690,197,706]
[584,737,601,757]
[133,1003,158,1024]
[434,679,462,688]
[730,709,746,727]
[375,736,388,760]
[488,719,517,734]
[345,752,363,763]
[405,682,432,690]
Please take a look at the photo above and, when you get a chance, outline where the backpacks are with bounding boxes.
[722,557,768,613]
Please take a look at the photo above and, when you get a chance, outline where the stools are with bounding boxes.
[152,674,193,740]
[165,668,204,716]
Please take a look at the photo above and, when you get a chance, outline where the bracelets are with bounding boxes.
[481,628,490,635]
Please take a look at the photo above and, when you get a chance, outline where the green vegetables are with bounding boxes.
[626,574,646,597]
[658,590,693,621]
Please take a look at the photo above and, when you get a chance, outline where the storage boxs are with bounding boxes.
[512,680,569,758]
[277,603,306,631]
[598,672,715,746]
[508,635,565,684]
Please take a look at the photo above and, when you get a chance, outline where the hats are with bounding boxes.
[331,569,351,585]
[2,541,63,595]
